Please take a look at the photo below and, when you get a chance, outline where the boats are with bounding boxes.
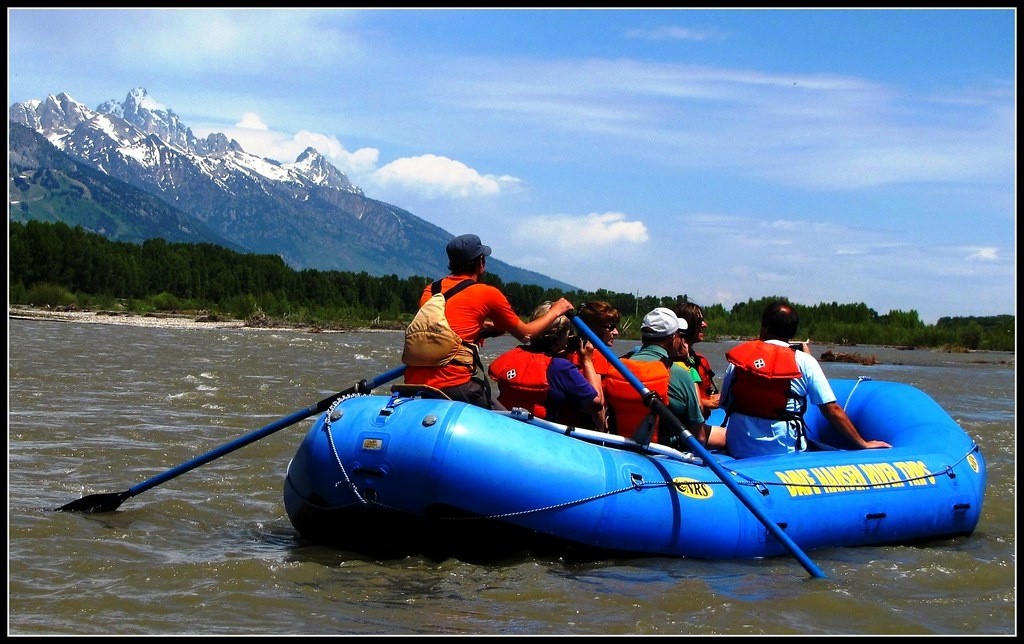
[283,375,987,564]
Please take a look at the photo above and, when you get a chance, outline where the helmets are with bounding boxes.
[761,300,800,337]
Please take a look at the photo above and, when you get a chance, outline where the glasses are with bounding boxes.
[597,323,617,331]
[694,318,705,324]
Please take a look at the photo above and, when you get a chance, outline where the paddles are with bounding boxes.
[567,311,827,579]
[54,325,498,515]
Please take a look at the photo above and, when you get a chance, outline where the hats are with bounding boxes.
[640,308,688,337]
[446,234,492,260]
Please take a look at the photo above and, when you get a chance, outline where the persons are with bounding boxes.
[401,234,575,410]
[719,301,891,459]
[487,300,604,428]
[673,303,721,419]
[605,307,727,453]
[554,299,621,432]
